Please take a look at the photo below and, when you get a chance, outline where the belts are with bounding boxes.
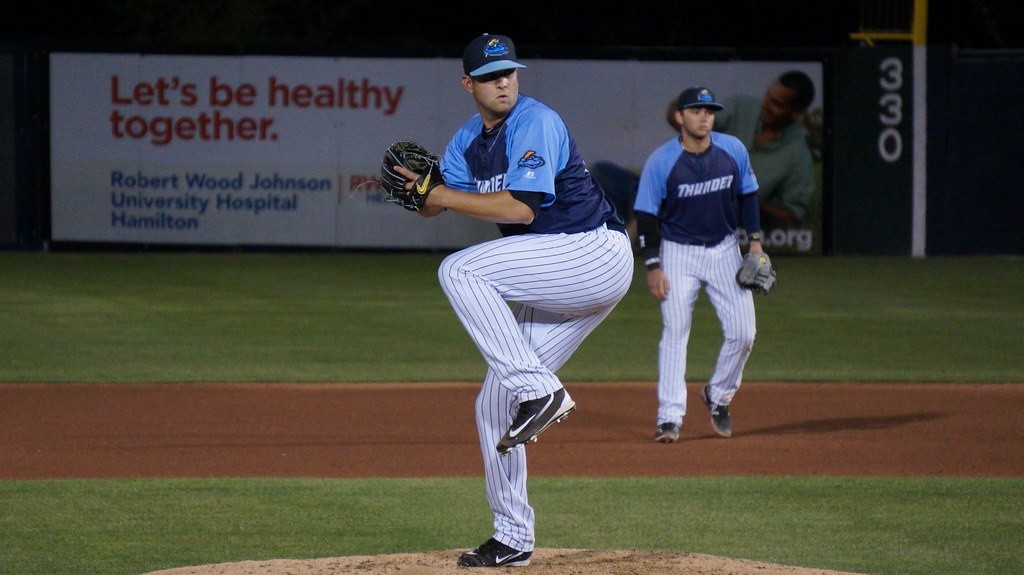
[606,221,629,239]
[675,232,733,248]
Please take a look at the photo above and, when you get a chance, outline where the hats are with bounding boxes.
[677,85,726,112]
[463,33,527,77]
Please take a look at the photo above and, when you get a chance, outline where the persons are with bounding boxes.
[378,35,635,566]
[589,68,817,258]
[633,84,777,442]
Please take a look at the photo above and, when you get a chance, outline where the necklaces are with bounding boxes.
[480,92,521,167]
[679,135,686,151]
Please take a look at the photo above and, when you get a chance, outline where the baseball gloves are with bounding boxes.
[379,139,446,214]
[734,252,777,295]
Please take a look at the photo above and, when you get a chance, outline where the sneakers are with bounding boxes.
[457,537,531,569]
[700,384,732,437]
[495,386,576,455]
[654,423,680,443]
